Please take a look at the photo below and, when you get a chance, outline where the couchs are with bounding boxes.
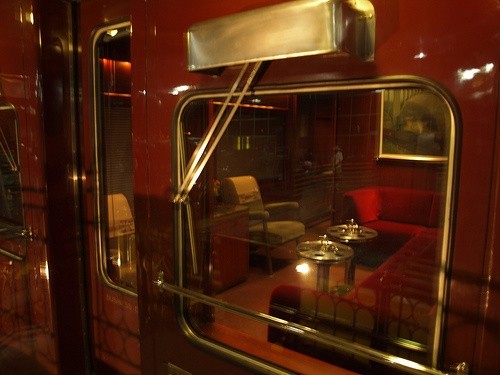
[268,179,451,374]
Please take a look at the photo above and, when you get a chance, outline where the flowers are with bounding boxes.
[211,178,221,197]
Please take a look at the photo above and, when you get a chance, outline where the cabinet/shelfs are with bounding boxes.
[211,201,253,295]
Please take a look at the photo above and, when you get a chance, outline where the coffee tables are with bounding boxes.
[293,239,355,296]
[323,222,379,289]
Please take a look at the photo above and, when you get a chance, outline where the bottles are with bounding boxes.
[321,234,329,251]
[349,219,359,234]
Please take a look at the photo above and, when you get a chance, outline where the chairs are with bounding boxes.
[221,172,308,277]
[107,192,139,289]
